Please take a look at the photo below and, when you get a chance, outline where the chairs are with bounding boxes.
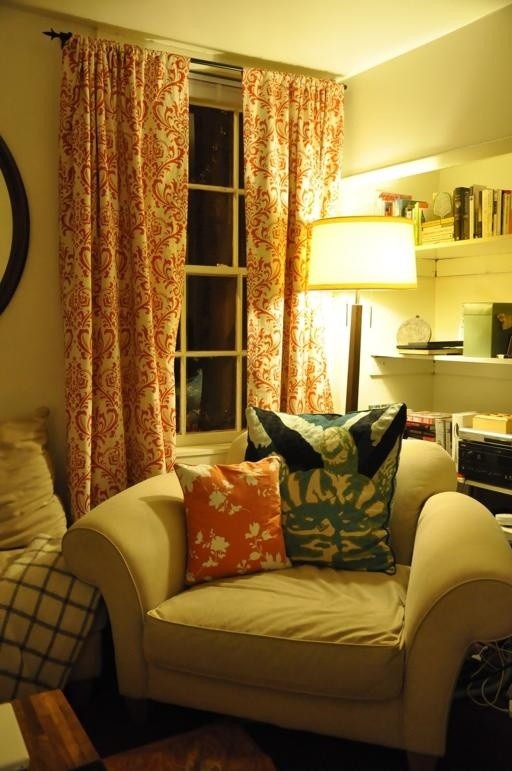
[61,426,512,771]
[2,535,102,696]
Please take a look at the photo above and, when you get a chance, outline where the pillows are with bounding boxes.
[0,407,67,550]
[174,455,292,585]
[244,404,408,575]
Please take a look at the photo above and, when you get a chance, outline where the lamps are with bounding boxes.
[306,217,417,414]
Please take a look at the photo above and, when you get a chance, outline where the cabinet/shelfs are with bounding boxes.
[332,134,511,513]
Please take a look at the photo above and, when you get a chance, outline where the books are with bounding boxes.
[385,184,512,247]
[367,403,489,474]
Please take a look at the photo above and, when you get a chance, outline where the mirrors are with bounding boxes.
[0,134,30,315]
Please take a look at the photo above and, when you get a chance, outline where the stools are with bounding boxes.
[0,689,105,771]
